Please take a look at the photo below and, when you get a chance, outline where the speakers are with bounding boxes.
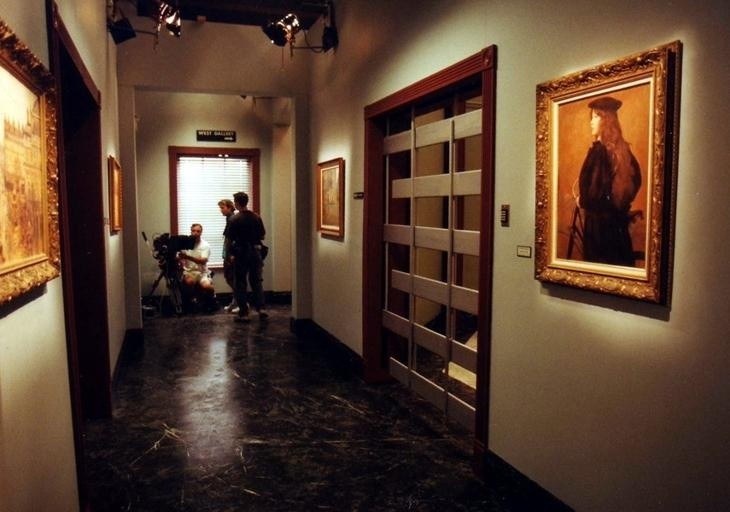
[109,18,136,44]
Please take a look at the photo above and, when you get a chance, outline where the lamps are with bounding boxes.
[262,11,337,58]
[106,0,182,54]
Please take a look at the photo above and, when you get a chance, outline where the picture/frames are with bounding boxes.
[109,155,124,232]
[316,158,343,237]
[535,40,681,307]
[0,16,61,307]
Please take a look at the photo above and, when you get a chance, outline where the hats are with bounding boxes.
[588,96,622,109]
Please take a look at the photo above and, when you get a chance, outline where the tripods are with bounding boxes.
[142,273,183,318]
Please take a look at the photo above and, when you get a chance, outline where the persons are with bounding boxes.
[574,96,643,267]
[177,224,216,315]
[223,192,269,318]
[218,200,241,313]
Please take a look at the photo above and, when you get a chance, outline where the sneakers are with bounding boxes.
[224,303,265,316]
